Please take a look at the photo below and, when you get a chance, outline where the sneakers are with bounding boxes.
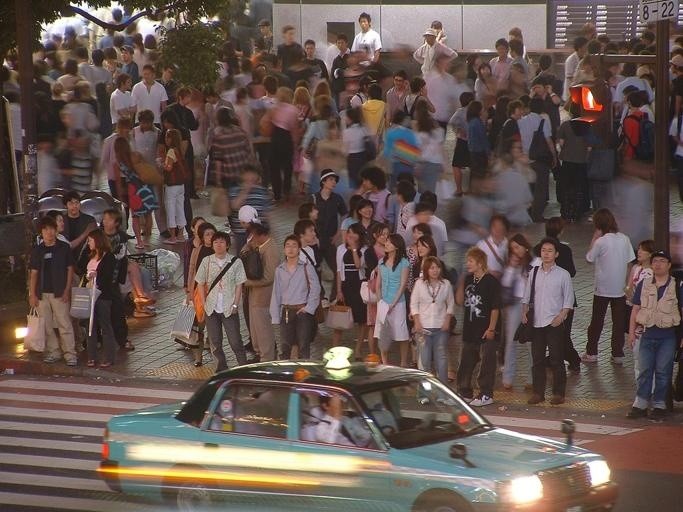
[63,353,79,367]
[418,396,431,407]
[609,354,626,366]
[98,359,114,368]
[525,392,546,406]
[132,294,157,305]
[40,349,63,364]
[131,308,159,319]
[567,355,583,373]
[445,396,474,409]
[85,357,97,368]
[624,406,649,419]
[435,398,449,406]
[544,353,554,369]
[468,393,496,407]
[523,382,534,391]
[119,340,136,352]
[502,381,514,391]
[647,407,671,424]
[577,352,599,363]
[550,395,567,406]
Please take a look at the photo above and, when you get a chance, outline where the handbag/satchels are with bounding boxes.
[623,297,634,336]
[22,302,48,355]
[167,296,204,353]
[528,128,554,164]
[312,300,327,326]
[132,161,169,188]
[257,120,276,138]
[512,307,535,344]
[207,185,235,218]
[68,269,103,317]
[323,296,357,334]
[391,139,425,169]
[237,249,265,284]
[296,119,308,138]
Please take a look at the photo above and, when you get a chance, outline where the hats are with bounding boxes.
[648,249,671,263]
[357,75,378,86]
[318,167,340,188]
[668,53,683,68]
[119,44,134,56]
[422,27,439,40]
[237,204,260,223]
[255,17,271,28]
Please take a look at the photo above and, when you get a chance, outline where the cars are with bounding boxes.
[96,345,620,511]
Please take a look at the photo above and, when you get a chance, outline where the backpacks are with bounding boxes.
[401,112,412,128]
[630,119,656,165]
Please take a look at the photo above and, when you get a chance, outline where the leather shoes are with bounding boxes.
[192,355,206,370]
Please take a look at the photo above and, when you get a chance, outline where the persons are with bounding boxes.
[314,395,372,447]
[0,2,682,421]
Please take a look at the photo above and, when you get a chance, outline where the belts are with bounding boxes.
[278,302,309,310]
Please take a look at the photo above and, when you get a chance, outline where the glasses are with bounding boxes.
[511,144,525,150]
